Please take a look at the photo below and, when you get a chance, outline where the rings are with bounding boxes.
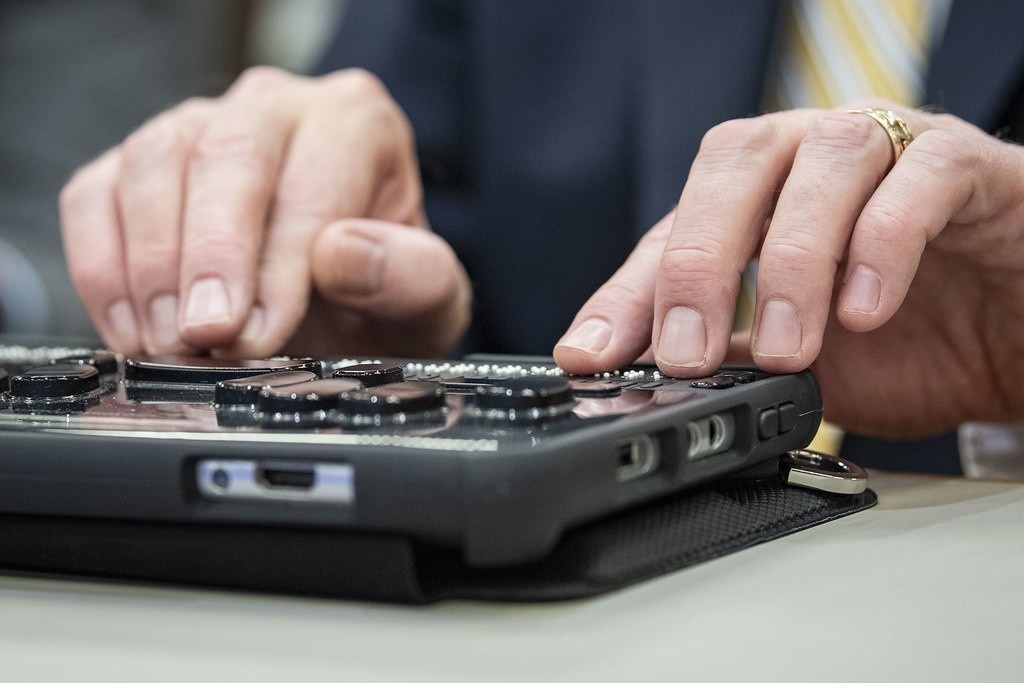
[850,97,915,160]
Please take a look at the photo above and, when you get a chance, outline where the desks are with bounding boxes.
[0,462,1024,683]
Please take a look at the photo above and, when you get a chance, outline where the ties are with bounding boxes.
[732,0,951,463]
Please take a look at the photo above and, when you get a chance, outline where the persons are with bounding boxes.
[45,1,1024,447]
